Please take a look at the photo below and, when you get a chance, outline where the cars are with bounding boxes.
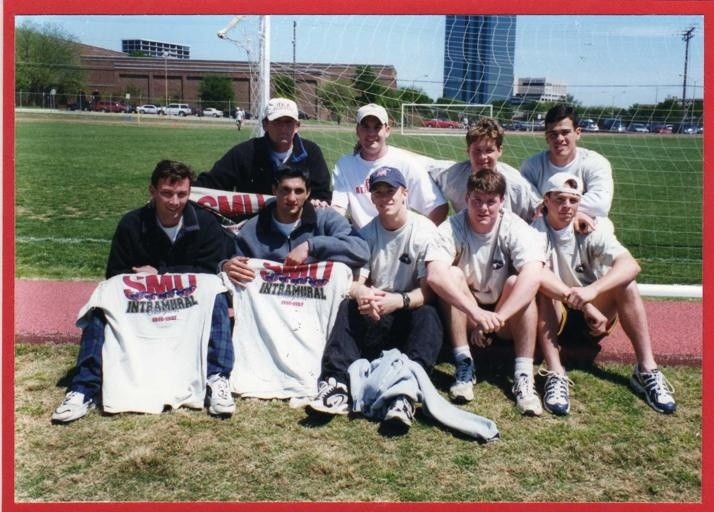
[49,92,227,118]
[415,113,702,136]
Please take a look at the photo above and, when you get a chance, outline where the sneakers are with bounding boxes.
[448,358,477,401]
[537,366,574,415]
[203,373,236,415]
[382,394,416,428]
[50,390,96,422]
[304,377,352,415]
[511,370,542,416]
[628,366,676,414]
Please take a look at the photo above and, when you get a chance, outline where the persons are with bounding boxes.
[518,100,614,218]
[529,172,678,415]
[235,105,243,130]
[311,103,449,234]
[463,114,468,131]
[305,166,439,428]
[190,97,332,205]
[216,165,372,289]
[51,159,238,422]
[429,117,596,235]
[425,170,546,416]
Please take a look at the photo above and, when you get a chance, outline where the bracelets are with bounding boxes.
[401,291,411,310]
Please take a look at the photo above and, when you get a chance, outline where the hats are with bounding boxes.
[263,97,298,122]
[545,172,584,198]
[355,103,388,126]
[369,166,406,192]
